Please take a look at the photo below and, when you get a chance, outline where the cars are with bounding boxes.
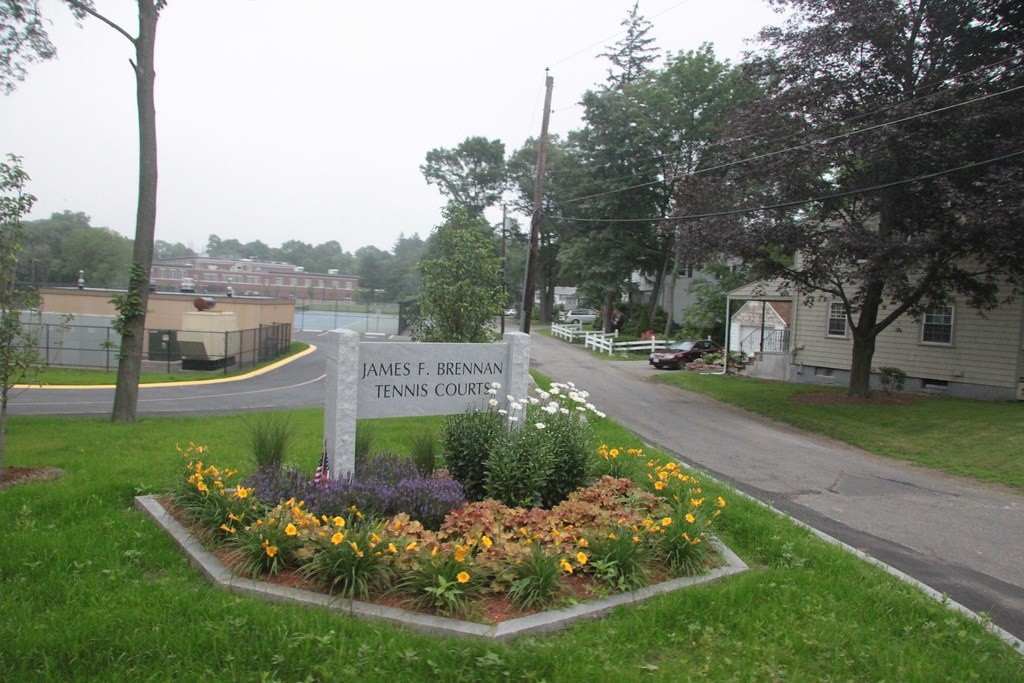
[559,307,597,325]
[504,308,518,316]
[648,339,723,369]
